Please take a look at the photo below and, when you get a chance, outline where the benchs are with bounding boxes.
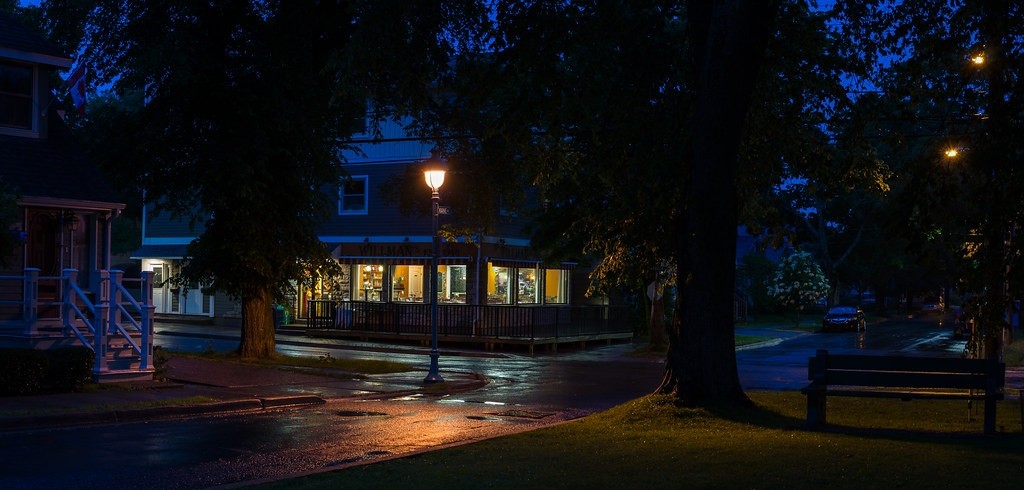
[802,350,1006,439]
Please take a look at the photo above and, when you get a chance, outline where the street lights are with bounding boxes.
[420,148,447,381]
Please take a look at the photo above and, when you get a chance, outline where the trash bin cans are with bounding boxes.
[275,305,290,328]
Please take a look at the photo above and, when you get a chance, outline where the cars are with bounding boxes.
[822,306,866,332]
[929,305,942,312]
[947,306,976,338]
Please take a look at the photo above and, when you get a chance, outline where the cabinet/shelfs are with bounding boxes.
[361,270,384,289]
